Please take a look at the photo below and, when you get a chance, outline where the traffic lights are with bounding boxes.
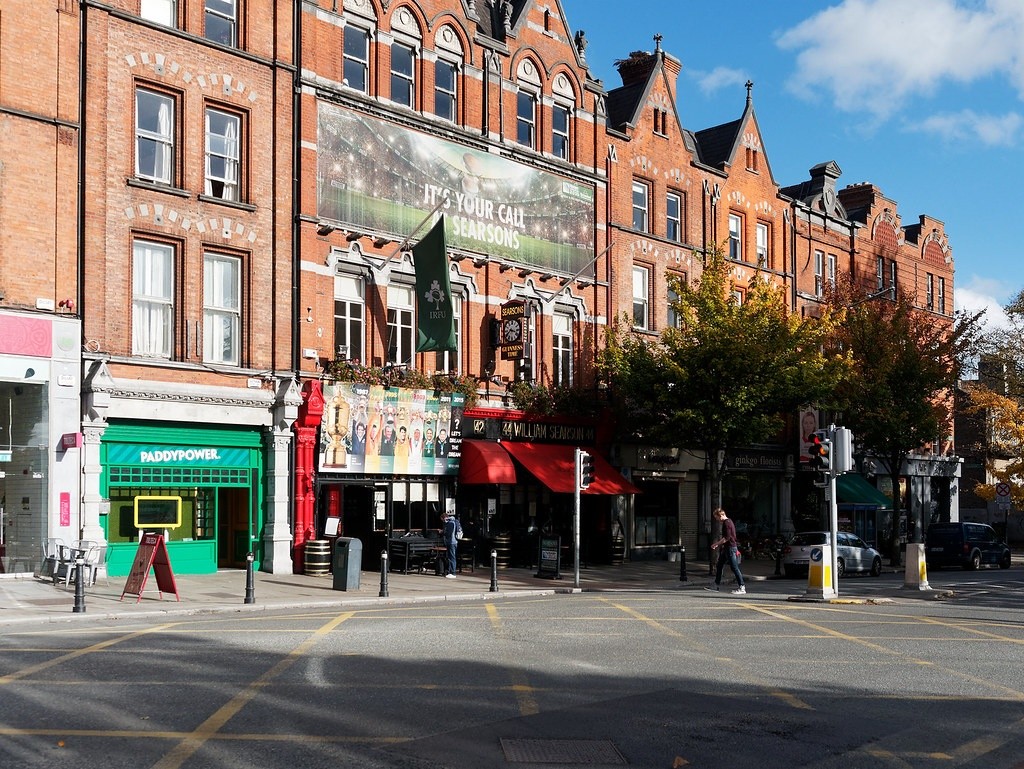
[580,453,595,489]
[835,428,856,472]
[815,441,833,472]
[807,431,829,486]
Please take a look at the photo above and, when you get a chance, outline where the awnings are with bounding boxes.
[827,474,893,505]
[460,441,640,494]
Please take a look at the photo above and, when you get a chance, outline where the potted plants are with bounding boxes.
[492,525,512,570]
[666,544,688,563]
[610,519,625,565]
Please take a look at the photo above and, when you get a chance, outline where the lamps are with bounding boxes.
[316,223,592,290]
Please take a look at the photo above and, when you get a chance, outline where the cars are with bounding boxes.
[782,530,882,579]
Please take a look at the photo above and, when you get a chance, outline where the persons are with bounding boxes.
[436,429,449,458]
[381,426,395,456]
[424,427,435,457]
[410,428,423,456]
[351,407,367,455]
[368,416,380,455]
[704,507,746,594]
[801,411,817,460]
[395,426,409,455]
[438,513,458,575]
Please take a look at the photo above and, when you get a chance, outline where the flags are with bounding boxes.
[412,215,457,353]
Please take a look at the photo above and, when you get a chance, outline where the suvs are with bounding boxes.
[925,522,1011,572]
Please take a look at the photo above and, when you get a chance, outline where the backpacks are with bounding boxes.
[451,519,464,539]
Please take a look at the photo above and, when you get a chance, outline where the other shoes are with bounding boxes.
[446,574,456,578]
[704,585,719,592]
[731,587,746,595]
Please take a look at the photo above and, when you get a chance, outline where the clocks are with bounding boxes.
[502,318,522,343]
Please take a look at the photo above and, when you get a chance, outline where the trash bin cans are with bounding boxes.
[332,537,363,592]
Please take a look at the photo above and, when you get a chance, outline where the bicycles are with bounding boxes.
[736,524,787,562]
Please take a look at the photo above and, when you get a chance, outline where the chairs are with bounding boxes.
[38,538,99,592]
[456,537,481,574]
